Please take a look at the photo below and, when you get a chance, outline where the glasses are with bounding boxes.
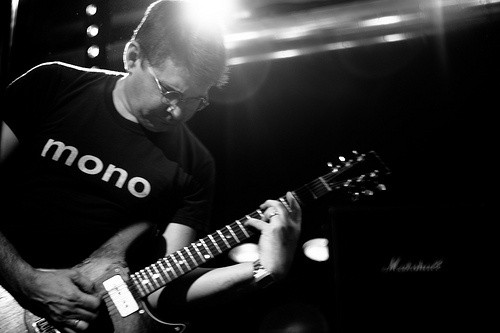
[147,62,209,112]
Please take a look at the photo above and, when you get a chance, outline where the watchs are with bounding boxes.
[252,259,277,292]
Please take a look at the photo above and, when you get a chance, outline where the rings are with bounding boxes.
[73,319,79,328]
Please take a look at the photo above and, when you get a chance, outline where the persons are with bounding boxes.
[0,0,302,333]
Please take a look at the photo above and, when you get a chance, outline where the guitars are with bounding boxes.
[0,146,397,332]
